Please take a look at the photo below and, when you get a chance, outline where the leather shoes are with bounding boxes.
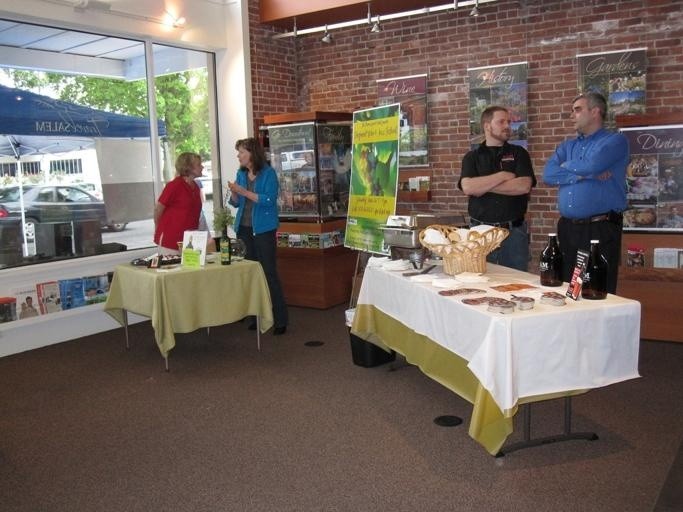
[248,322,258,331]
[274,327,286,336]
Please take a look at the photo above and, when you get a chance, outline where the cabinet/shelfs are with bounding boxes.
[257,110,358,310]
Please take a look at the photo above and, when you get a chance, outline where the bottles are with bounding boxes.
[184,235,195,251]
[217,223,231,267]
[538,231,561,287]
[581,238,610,300]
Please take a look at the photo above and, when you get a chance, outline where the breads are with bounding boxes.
[448,232,461,244]
[469,231,481,241]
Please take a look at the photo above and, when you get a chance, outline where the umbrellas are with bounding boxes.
[0,83,166,258]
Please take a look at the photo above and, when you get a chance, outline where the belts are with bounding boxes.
[563,213,609,224]
[470,219,523,229]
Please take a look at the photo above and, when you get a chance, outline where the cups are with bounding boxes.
[412,259,424,270]
[204,239,217,264]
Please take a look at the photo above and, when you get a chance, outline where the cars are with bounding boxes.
[0,183,129,243]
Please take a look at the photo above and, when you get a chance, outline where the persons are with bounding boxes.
[542,92,630,295]
[664,205,683,228]
[153,153,204,257]
[457,107,537,273]
[227,138,289,336]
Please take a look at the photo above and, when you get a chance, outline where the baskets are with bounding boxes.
[419,223,510,276]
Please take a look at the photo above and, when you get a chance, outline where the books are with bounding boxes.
[398,176,430,192]
[180,230,208,266]
[276,228,345,248]
[0,272,116,325]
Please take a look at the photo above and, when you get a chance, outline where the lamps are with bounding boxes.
[370,23,383,33]
[321,33,332,44]
[470,6,480,17]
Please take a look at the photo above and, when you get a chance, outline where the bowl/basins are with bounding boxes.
[488,299,515,315]
[540,291,568,305]
[512,296,535,309]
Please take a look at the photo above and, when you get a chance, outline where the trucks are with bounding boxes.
[269,150,315,171]
